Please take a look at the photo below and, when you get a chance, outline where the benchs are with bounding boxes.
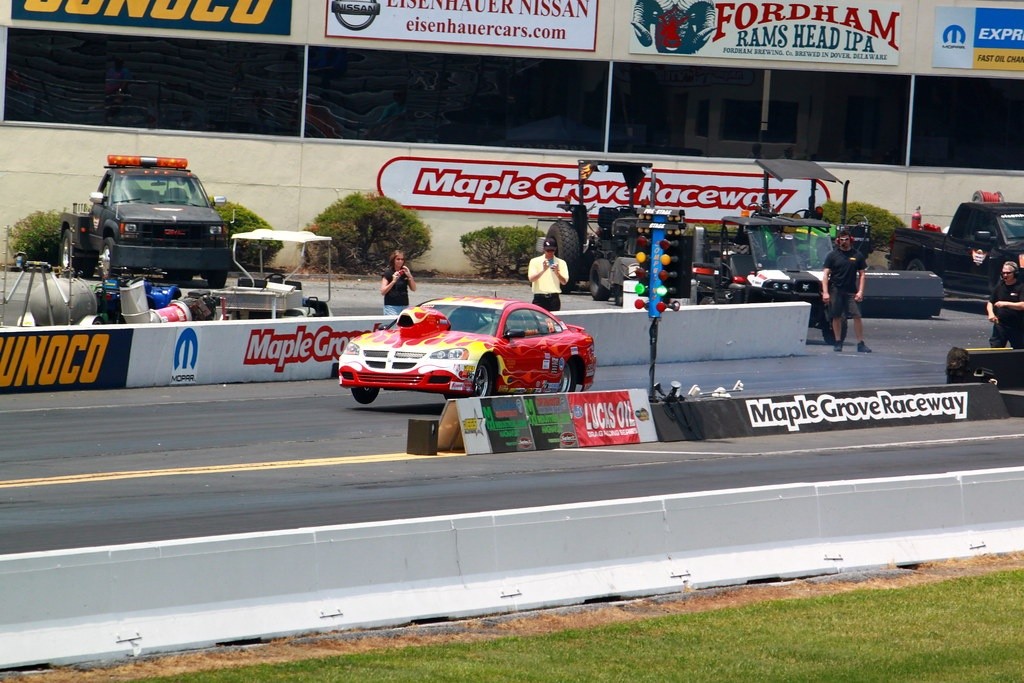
[271,279,302,295]
[729,251,779,280]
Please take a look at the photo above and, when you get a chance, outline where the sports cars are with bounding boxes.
[339,297,597,405]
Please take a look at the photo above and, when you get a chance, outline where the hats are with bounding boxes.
[544,238,558,251]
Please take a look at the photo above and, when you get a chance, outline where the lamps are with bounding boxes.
[654,379,744,402]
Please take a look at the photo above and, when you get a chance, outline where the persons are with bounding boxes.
[381,250,416,315]
[986,262,1024,349]
[946,348,997,387]
[528,238,569,311]
[822,230,873,353]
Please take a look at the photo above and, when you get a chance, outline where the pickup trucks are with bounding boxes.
[58,156,235,291]
[690,213,847,346]
[884,200,1024,299]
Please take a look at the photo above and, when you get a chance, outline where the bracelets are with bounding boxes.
[408,276,414,280]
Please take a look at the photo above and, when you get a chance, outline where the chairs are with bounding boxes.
[599,206,623,240]
[164,187,187,200]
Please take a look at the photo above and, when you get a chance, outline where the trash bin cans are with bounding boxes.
[624,278,649,312]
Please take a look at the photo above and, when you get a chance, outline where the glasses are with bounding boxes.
[1002,272,1013,274]
[840,237,849,241]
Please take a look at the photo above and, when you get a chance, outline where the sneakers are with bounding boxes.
[834,340,843,351]
[858,341,872,352]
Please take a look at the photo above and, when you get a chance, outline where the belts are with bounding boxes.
[534,294,558,298]
